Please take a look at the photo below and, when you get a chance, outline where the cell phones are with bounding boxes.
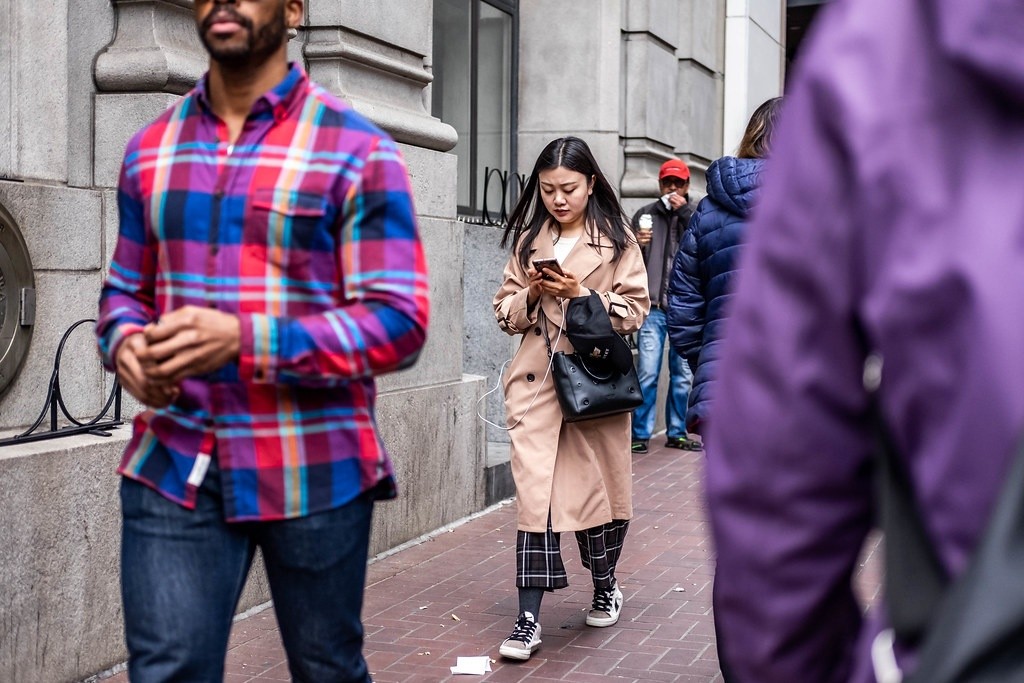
[532,258,565,282]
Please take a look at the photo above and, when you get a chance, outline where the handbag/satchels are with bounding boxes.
[549,350,645,423]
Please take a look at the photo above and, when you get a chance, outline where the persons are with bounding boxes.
[631,159,703,454]
[665,96,786,439]
[709,0,1024,683]
[95,1,429,683]
[494,137,652,661]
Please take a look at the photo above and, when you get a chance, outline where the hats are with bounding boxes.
[659,160,690,180]
[567,289,634,377]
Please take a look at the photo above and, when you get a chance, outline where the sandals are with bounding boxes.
[632,439,648,453]
[665,436,702,452]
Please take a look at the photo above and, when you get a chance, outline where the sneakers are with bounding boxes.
[586,580,623,627]
[500,612,542,661]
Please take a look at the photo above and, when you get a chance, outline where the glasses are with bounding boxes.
[661,178,688,188]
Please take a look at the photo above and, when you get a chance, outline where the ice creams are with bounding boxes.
[639,214,653,232]
